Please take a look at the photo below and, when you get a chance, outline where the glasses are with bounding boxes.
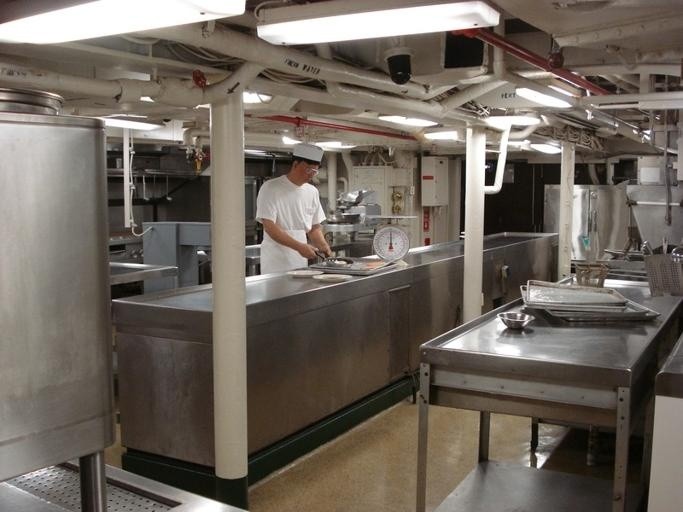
[302,163,317,176]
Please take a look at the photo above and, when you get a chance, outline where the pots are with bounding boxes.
[313,250,355,269]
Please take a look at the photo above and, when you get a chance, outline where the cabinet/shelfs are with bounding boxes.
[352,166,393,217]
[421,156,449,206]
[543,184,631,263]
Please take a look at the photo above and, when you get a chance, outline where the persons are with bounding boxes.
[254,145,333,275]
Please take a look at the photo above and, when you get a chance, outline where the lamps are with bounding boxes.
[0,0,246,46]
[104,116,165,132]
[253,0,502,47]
[516,84,573,109]
[548,78,583,99]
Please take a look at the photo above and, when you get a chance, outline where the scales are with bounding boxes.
[367,215,417,265]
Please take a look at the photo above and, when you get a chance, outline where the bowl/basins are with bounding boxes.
[497,312,535,331]
[329,212,360,224]
[0,88,67,118]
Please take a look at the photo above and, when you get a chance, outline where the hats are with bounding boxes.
[292,144,323,162]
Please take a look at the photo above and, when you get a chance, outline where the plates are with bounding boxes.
[311,275,354,285]
[286,269,323,279]
